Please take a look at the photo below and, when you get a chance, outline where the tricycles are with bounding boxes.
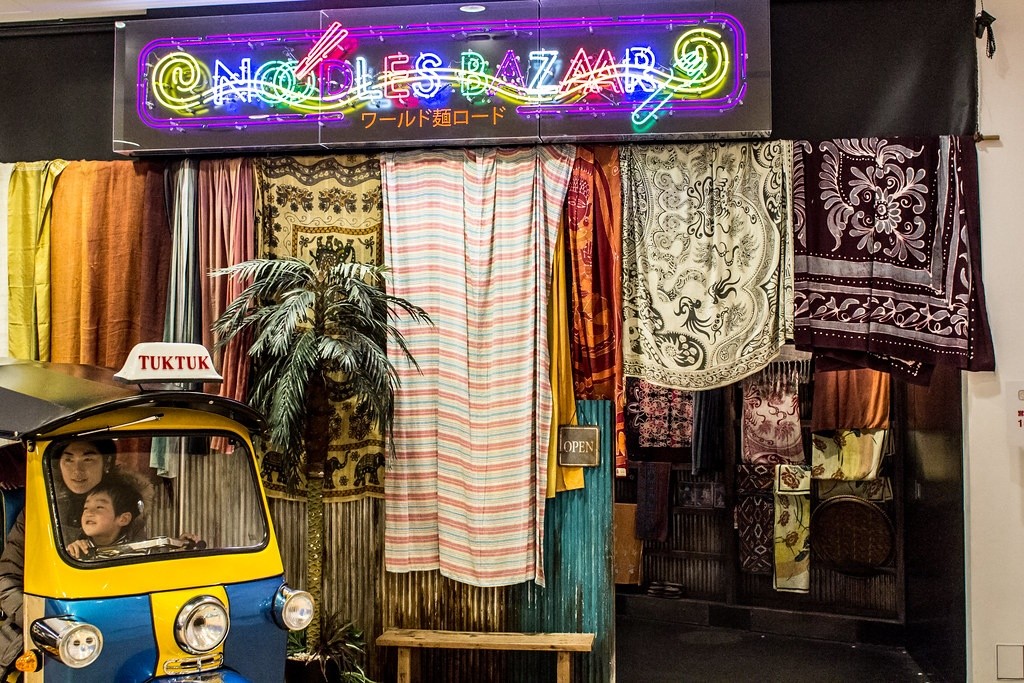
[0,342,315,683]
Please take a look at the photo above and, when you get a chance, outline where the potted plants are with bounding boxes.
[205,257,435,683]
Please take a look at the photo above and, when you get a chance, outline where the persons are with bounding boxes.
[1,433,117,683]
[66,479,203,559]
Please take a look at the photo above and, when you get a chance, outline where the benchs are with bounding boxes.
[376,627,595,683]
